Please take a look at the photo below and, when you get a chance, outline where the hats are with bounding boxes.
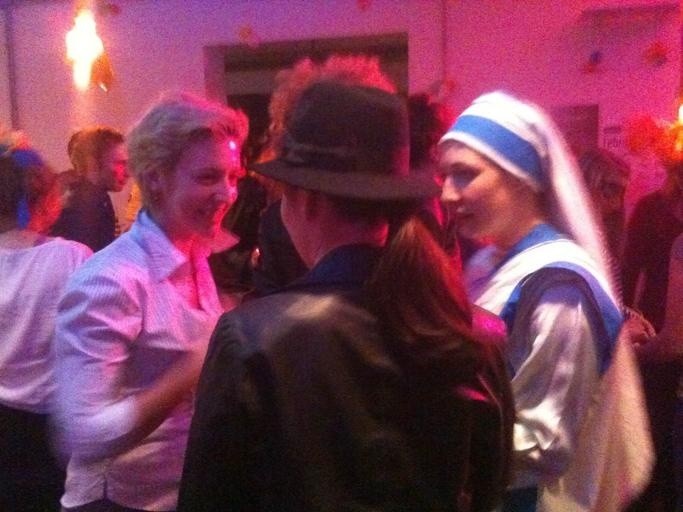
[244,81,442,203]
[436,89,655,512]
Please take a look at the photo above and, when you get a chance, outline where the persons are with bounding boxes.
[621,121,683,511]
[0,53,654,512]
